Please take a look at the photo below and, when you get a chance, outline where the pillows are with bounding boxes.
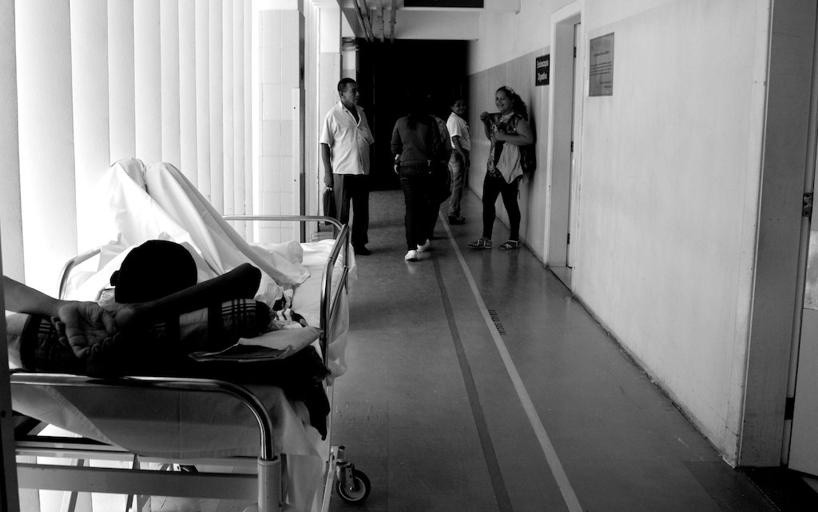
[18,295,277,376]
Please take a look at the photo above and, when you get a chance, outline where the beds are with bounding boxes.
[9,215,356,512]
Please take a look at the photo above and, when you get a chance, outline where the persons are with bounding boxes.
[3,241,198,359]
[320,78,376,256]
[391,85,533,260]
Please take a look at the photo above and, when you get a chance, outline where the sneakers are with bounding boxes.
[353,245,371,256]
[468,238,492,249]
[498,240,520,250]
[405,249,417,261]
[448,215,466,224]
[417,239,431,251]
